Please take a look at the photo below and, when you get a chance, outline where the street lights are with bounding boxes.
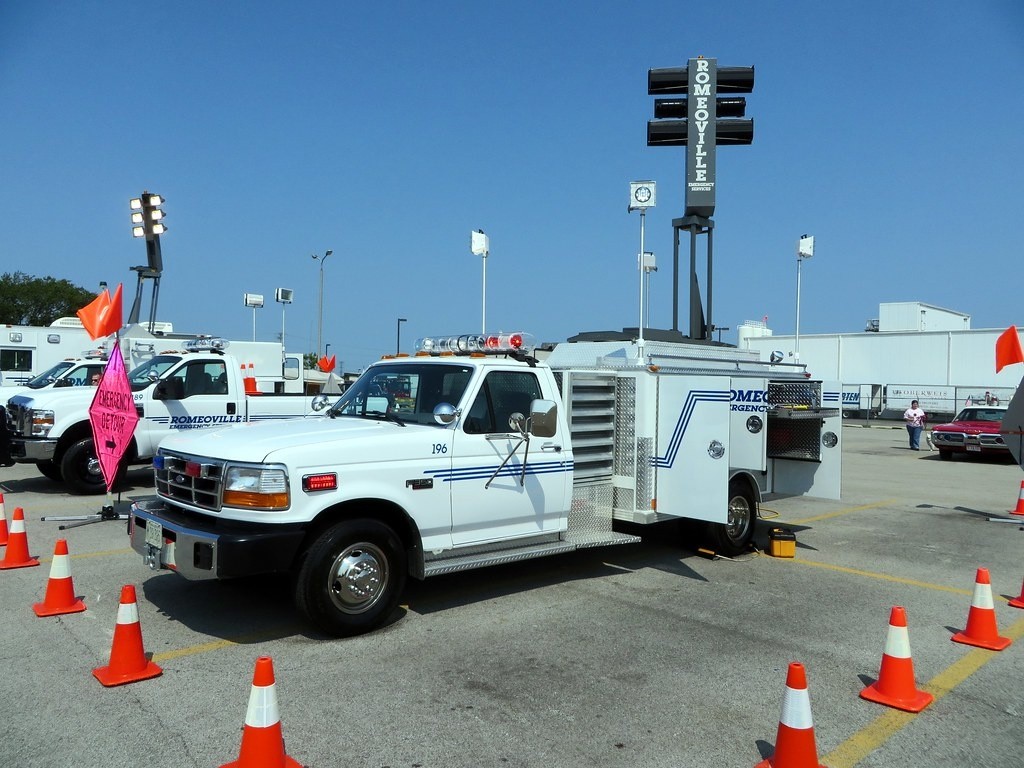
[311,250,333,363]
[325,344,331,357]
[397,318,407,354]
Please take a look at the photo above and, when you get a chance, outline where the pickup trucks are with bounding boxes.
[21,333,396,495]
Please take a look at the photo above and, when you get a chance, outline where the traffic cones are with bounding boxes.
[32,540,86,617]
[240,363,262,395]
[0,507,40,570]
[753,661,829,768]
[1009,480,1024,515]
[89,584,163,687]
[0,492,9,546]
[215,656,304,768]
[950,567,1013,651]
[858,605,934,714]
[1008,577,1024,609]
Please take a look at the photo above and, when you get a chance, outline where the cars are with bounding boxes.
[931,406,1014,465]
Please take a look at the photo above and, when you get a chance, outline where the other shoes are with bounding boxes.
[911,446,920,451]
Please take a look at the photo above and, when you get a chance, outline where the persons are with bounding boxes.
[92,372,102,386]
[904,400,926,451]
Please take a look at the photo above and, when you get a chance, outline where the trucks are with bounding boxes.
[0,189,305,468]
[133,178,843,649]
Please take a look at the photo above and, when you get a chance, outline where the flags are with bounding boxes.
[104,284,122,337]
[995,325,1024,374]
[327,355,335,371]
[317,356,329,371]
[76,289,111,342]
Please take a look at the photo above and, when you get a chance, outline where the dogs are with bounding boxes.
[926,433,935,451]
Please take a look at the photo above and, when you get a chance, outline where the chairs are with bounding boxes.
[494,392,532,433]
[208,373,227,393]
[190,374,212,394]
[421,389,462,414]
